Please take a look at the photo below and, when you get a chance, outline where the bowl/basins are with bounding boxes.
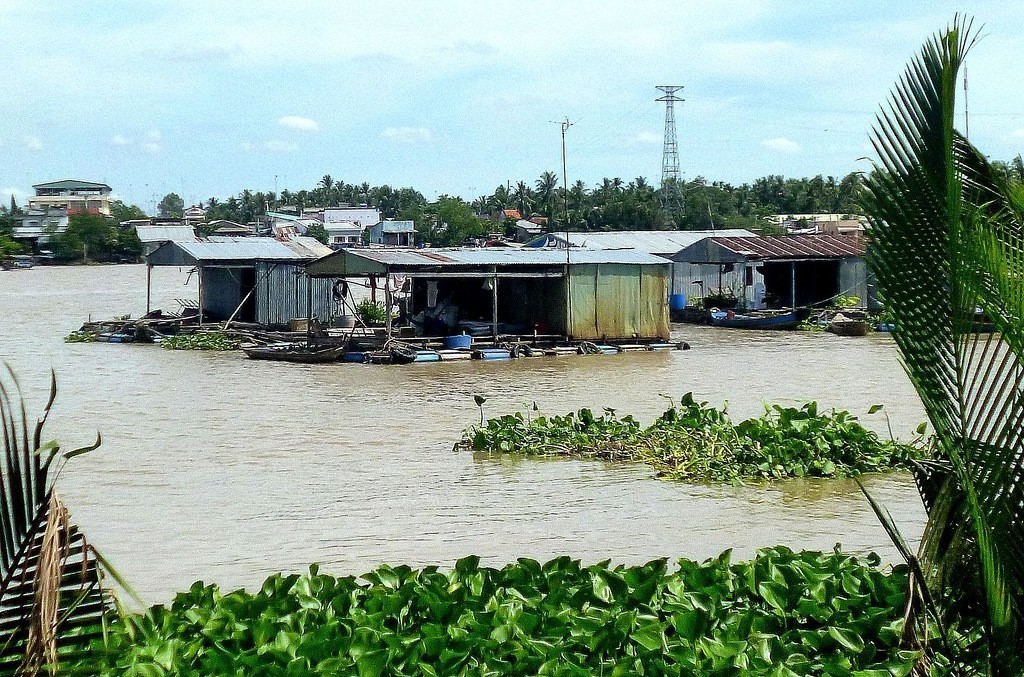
[444,335,471,350]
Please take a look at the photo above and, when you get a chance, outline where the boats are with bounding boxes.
[941,299,1006,333]
[516,218,547,234]
[522,235,549,247]
[703,285,738,309]
[242,333,345,363]
[829,312,869,336]
[709,306,809,330]
[391,347,418,364]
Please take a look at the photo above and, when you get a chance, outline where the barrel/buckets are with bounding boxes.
[671,294,686,310]
